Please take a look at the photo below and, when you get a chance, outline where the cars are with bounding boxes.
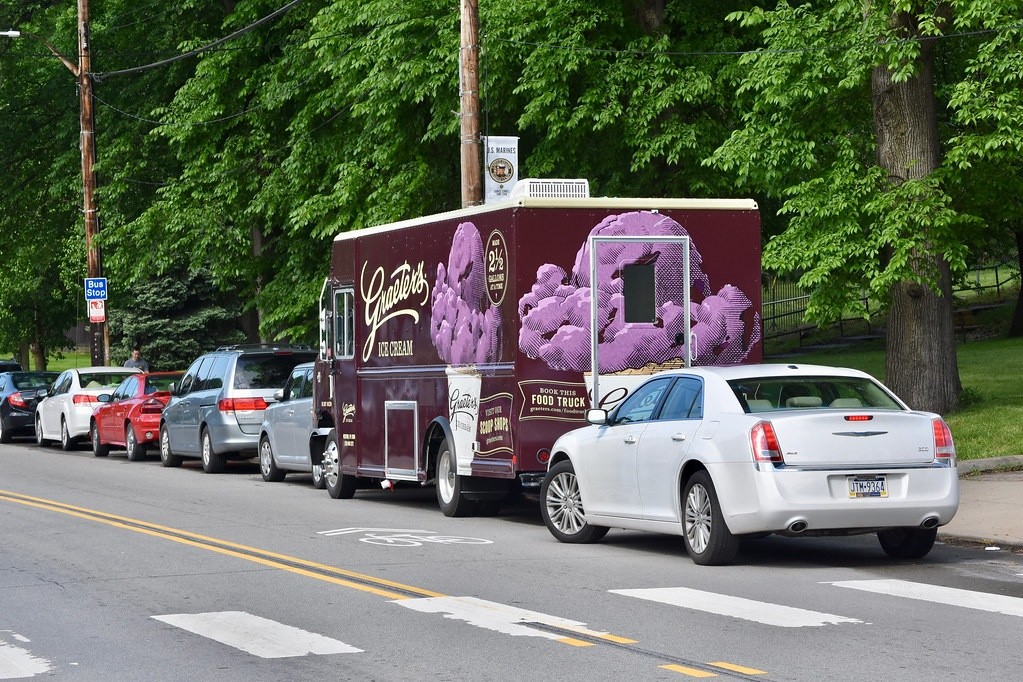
[0,370,64,444]
[90,369,188,461]
[35,367,160,450]
[540,361,959,567]
[257,362,327,490]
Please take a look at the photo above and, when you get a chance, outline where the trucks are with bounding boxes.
[310,179,760,518]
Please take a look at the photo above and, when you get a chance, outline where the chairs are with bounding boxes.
[746,398,864,413]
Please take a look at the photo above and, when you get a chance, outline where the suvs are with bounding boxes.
[0,359,32,387]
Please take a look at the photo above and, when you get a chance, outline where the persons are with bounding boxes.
[124,346,149,373]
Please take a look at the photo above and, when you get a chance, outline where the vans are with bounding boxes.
[158,342,318,473]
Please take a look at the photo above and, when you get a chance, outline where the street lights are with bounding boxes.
[0,29,107,368]
